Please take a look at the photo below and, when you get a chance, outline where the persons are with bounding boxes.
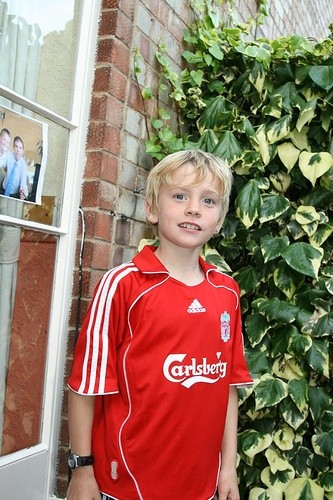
[66,150,253,500]
[0,127,30,201]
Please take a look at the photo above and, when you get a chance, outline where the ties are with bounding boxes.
[4,162,19,197]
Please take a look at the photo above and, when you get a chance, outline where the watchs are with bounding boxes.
[68,455,95,471]
